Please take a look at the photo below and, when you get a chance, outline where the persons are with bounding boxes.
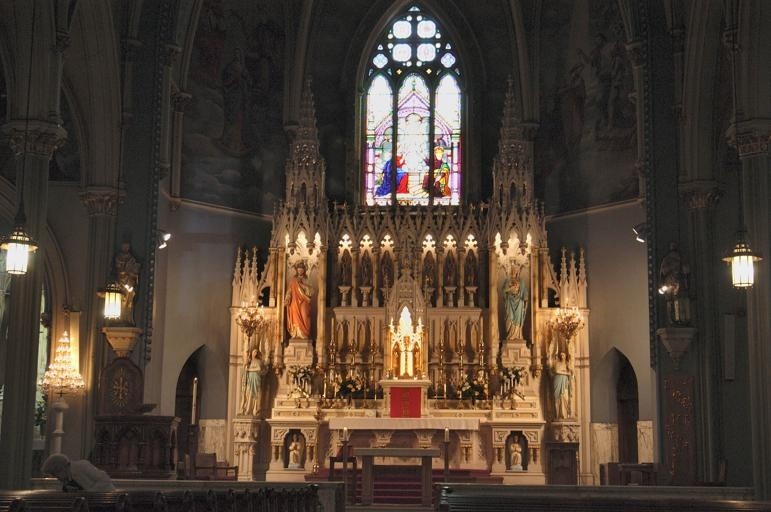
[553,351,572,419]
[284,263,313,339]
[214,48,251,157]
[510,436,523,470]
[239,348,263,416]
[288,434,302,469]
[42,454,115,489]
[577,33,626,128]
[502,265,528,340]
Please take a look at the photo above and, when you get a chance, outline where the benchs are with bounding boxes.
[438,484,771,510]
[1,483,325,511]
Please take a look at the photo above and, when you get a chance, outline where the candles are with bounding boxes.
[343,424,348,441]
[444,426,449,441]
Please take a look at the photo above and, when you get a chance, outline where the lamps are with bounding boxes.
[38,328,85,399]
[723,0,763,289]
[1,0,39,275]
[100,0,126,320]
